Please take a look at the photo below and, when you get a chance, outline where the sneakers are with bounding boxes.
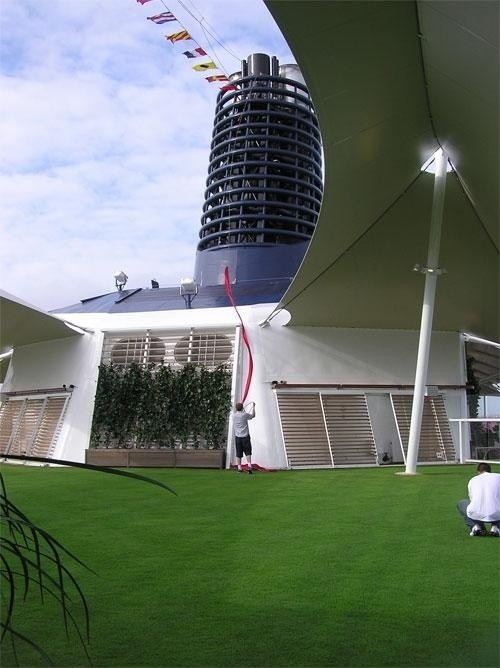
[489,527,500,536]
[247,467,252,474]
[469,523,482,536]
[237,468,243,473]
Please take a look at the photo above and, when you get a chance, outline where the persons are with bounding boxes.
[231,402,255,474]
[456,463,500,536]
